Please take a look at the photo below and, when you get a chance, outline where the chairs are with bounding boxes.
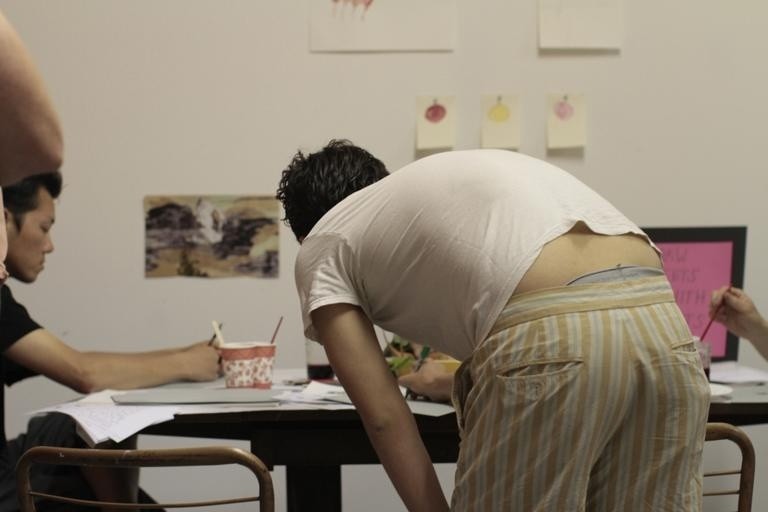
[13,446,275,512]
[699,423,754,512]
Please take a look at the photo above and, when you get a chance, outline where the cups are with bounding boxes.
[218,343,278,389]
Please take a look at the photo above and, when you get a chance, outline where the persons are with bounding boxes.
[708,285,768,362]
[0,6,68,288]
[273,137,713,509]
[0,166,221,510]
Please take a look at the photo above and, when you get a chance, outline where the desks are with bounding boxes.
[25,370,768,512]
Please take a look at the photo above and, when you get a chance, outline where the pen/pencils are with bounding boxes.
[701,284,733,341]
[209,324,224,345]
[269,316,285,344]
[405,346,430,398]
